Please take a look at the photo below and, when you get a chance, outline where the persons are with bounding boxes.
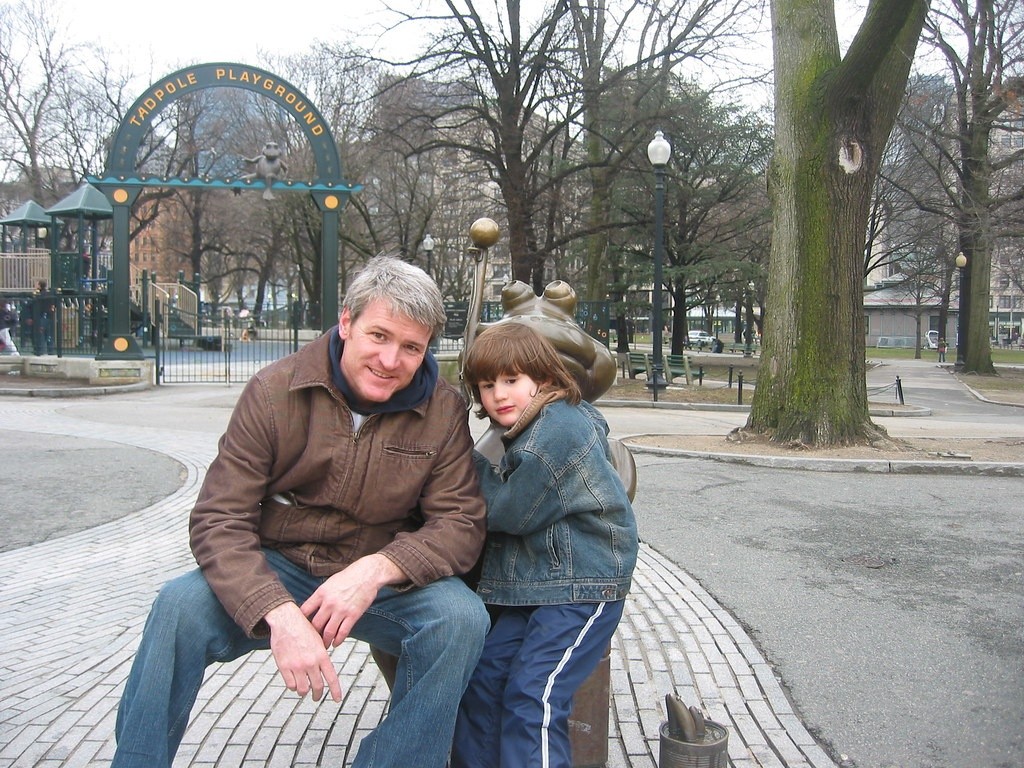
[0,278,54,356]
[239,304,258,341]
[109,252,492,768]
[82,248,108,351]
[446,321,637,768]
[938,338,946,363]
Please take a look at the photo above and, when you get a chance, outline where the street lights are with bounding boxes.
[423,234,435,277]
[954,251,967,373]
[643,130,672,393]
[713,295,720,339]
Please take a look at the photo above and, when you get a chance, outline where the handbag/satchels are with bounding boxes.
[945,346,948,353]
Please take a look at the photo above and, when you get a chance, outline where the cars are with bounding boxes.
[686,330,715,343]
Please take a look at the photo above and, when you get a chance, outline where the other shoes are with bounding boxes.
[11,352,19,356]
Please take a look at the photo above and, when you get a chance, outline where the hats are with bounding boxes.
[940,338,943,340]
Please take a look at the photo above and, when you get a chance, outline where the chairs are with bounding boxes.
[626,352,705,387]
